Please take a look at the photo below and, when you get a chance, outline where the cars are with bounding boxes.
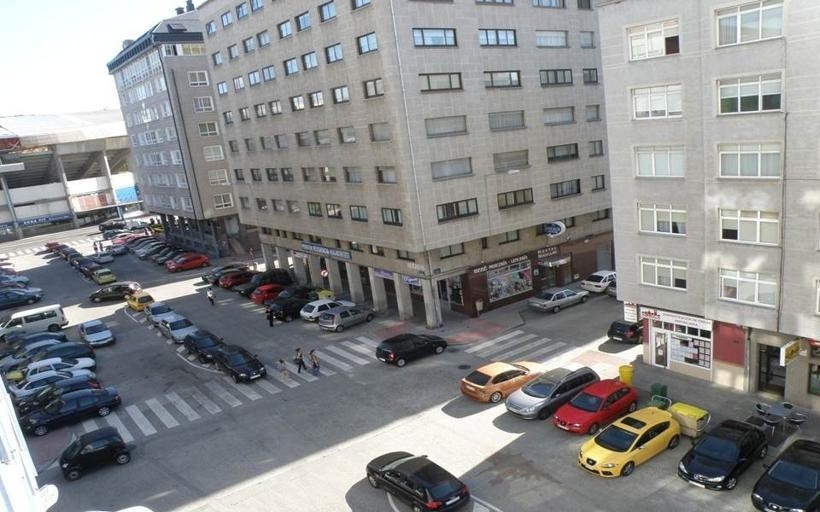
[751,439,819,512]
[526,286,590,314]
[201,259,318,307]
[299,298,357,324]
[676,419,769,493]
[581,270,617,294]
[128,290,268,384]
[552,378,640,439]
[0,252,135,482]
[607,279,616,299]
[459,360,549,403]
[576,405,682,479]
[365,451,470,511]
[43,217,210,290]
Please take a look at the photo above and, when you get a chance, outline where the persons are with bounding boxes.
[308,349,321,376]
[207,288,217,305]
[265,305,274,328]
[99,241,103,251]
[279,360,289,383]
[293,347,308,373]
[92,242,98,253]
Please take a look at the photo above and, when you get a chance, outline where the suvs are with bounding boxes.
[270,296,311,322]
[318,306,374,333]
[606,316,643,345]
[376,331,447,367]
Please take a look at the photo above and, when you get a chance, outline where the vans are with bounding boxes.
[504,360,601,421]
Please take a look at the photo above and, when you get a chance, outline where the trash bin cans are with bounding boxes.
[619,365,633,385]
